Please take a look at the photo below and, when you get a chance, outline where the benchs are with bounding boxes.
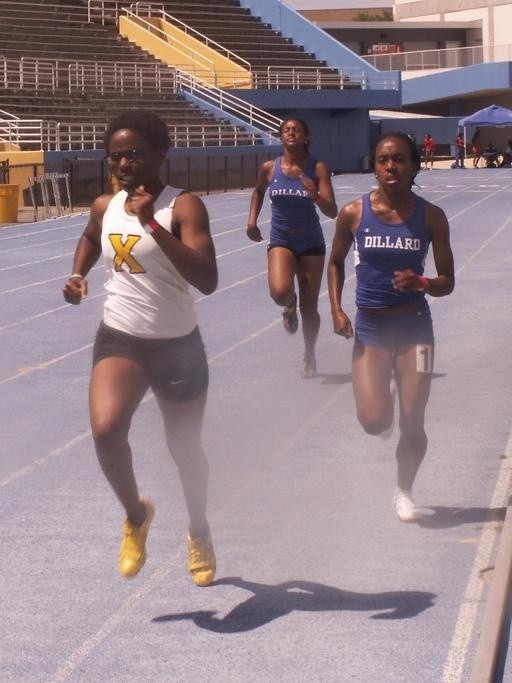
[1,0,362,152]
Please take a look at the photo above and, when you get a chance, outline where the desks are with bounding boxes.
[0,184,19,223]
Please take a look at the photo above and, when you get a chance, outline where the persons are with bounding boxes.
[484,142,500,165]
[245,116,339,380]
[455,130,465,169]
[499,140,511,169]
[327,132,456,523]
[63,108,219,590]
[422,132,437,171]
[472,131,481,169]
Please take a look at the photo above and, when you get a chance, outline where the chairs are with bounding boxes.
[0,158,9,184]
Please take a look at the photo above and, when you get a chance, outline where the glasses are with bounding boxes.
[110,151,140,161]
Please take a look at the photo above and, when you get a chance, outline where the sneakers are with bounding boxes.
[283,293,298,334]
[118,497,155,579]
[381,385,395,438]
[393,494,421,521]
[187,527,216,587]
[302,357,316,378]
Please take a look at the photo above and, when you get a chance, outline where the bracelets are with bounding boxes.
[141,214,162,235]
[67,274,84,282]
[315,190,321,205]
[419,276,429,295]
